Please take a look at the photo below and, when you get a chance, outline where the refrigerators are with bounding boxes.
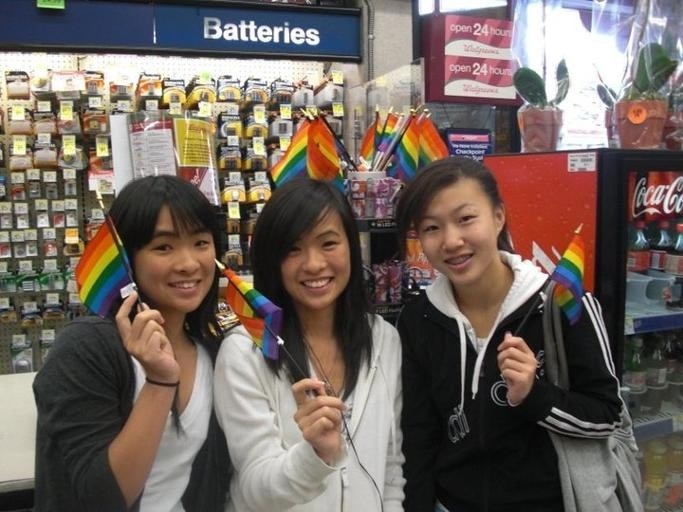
[482,150,683,511]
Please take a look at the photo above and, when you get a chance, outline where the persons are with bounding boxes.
[212,177,408,512]
[393,153,623,511]
[30,174,234,512]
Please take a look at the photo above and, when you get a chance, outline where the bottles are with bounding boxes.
[622,219,683,512]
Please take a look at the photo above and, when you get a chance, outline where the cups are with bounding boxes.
[346,178,399,217]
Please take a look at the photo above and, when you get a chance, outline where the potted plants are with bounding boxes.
[508,41,683,155]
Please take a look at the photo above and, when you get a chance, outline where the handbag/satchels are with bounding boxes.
[540,276,647,512]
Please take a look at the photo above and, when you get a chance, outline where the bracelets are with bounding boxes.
[145,374,180,388]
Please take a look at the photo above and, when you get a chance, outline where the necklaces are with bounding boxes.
[307,345,340,390]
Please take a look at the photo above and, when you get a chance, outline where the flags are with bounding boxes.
[548,233,585,330]
[71,213,132,319]
[222,266,284,363]
[266,106,450,193]
